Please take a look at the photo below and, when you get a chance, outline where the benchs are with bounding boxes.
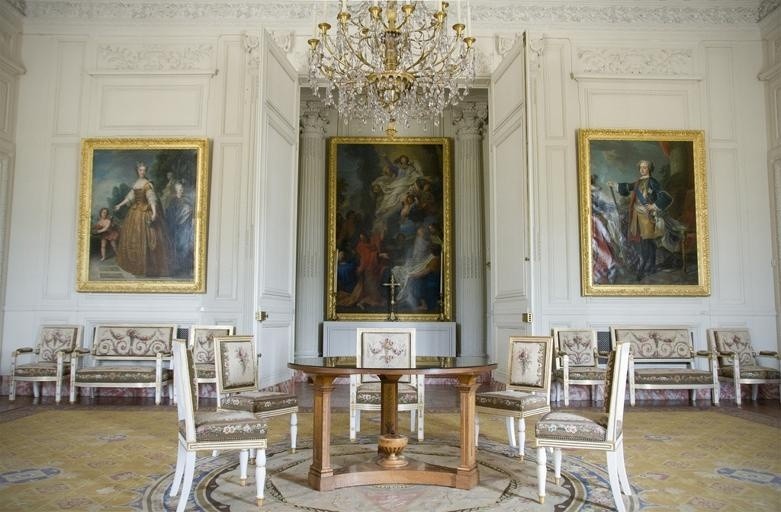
[610,325,718,406]
[74,324,177,408]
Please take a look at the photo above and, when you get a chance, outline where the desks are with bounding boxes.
[293,357,497,491]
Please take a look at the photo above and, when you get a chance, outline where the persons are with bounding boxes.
[94,159,194,280]
[607,159,673,273]
[337,153,449,311]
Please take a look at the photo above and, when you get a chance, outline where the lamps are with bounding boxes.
[304,0,477,139]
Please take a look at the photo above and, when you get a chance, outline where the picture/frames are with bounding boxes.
[78,138,211,295]
[577,130,713,299]
[322,135,454,324]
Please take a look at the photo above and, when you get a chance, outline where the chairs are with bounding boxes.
[351,327,428,443]
[473,336,555,457]
[550,326,613,409]
[708,322,779,408]
[9,324,85,403]
[186,325,237,411]
[169,339,267,511]
[215,336,300,454]
[534,342,634,512]
